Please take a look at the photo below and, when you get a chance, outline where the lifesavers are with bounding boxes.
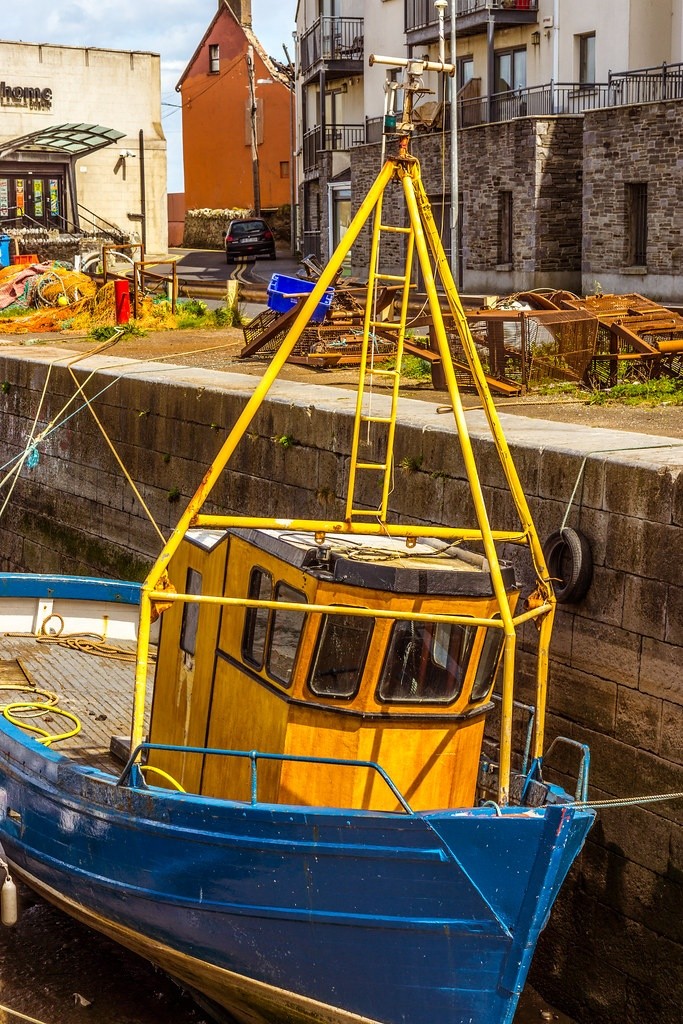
[542,527,593,604]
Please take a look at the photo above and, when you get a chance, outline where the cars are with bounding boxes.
[222,218,277,264]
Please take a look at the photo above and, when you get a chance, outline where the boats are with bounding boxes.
[0,53,597,1024]
